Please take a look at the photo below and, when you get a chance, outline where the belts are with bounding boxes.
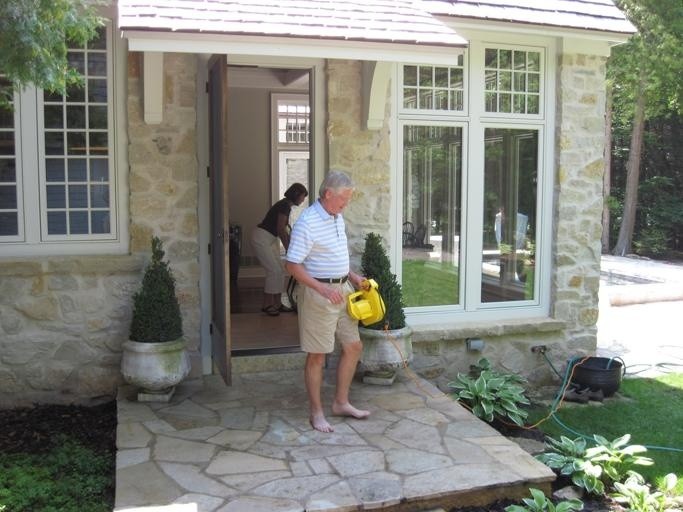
[313,275,348,284]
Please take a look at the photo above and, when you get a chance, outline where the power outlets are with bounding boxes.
[531,345,546,353]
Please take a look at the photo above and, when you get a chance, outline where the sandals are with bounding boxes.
[261,303,294,317]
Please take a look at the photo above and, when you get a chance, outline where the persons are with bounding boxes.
[284,171,370,434]
[249,183,308,316]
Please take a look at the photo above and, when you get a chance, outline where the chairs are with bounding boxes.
[402,221,415,248]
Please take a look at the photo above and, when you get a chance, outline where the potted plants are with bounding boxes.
[121,230,192,404]
[355,232,414,386]
